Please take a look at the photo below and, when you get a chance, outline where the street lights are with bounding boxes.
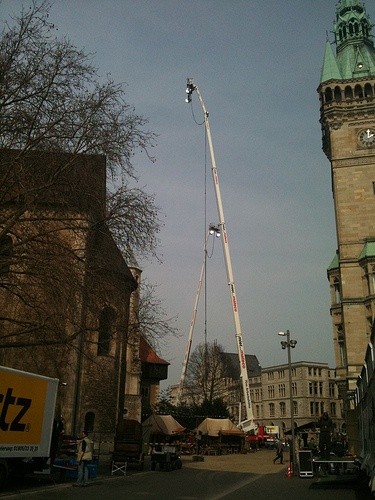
[277,330,299,478]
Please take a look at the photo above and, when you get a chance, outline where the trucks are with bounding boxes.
[1,365,60,488]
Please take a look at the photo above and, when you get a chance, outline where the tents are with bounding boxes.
[196,417,240,436]
[141,414,183,435]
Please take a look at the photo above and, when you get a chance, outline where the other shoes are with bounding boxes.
[273,460,275,464]
[280,462,283,464]
[321,448,330,455]
[82,485,89,487]
[72,484,79,487]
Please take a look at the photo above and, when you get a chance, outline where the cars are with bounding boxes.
[151,452,183,471]
[58,434,83,460]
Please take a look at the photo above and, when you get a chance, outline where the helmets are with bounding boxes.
[323,412,328,416]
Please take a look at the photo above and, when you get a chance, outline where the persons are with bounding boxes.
[273,441,285,464]
[72,431,92,486]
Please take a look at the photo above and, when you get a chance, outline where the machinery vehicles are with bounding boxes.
[142,77,291,454]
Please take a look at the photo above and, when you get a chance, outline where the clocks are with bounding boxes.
[354,126,375,149]
